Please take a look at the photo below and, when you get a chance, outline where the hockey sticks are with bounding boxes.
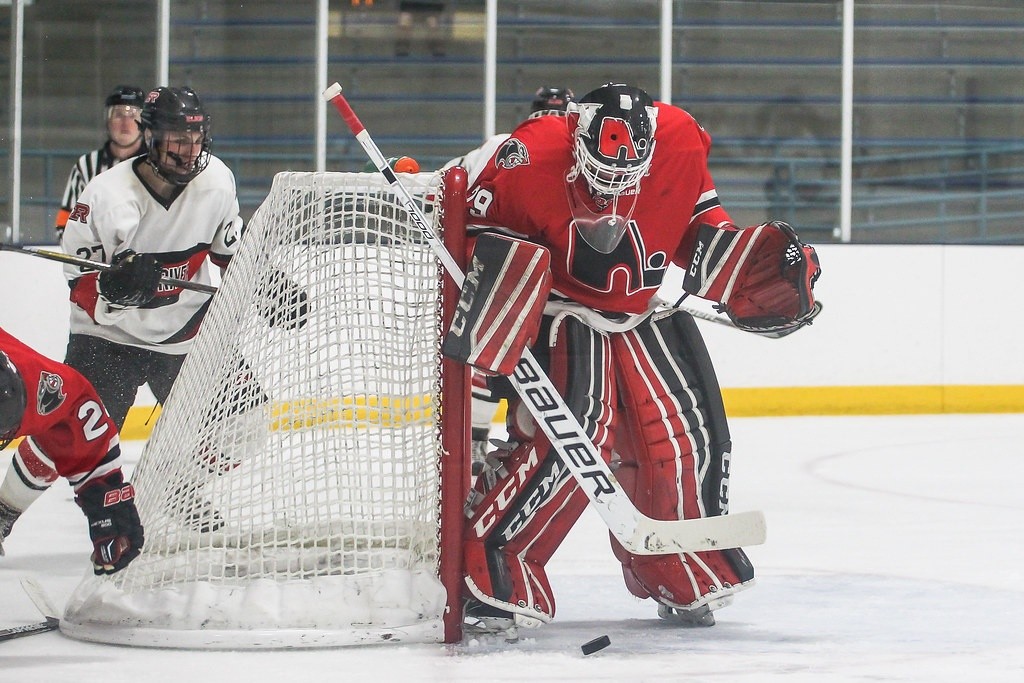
[0,575,61,641]
[0,240,281,309]
[324,81,768,555]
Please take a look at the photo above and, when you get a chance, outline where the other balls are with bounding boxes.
[580,635,613,656]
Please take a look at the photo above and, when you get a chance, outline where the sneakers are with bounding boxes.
[461,599,519,644]
[655,598,715,626]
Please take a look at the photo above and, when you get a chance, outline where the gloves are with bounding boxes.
[100,249,163,306]
[74,483,144,575]
[252,270,311,330]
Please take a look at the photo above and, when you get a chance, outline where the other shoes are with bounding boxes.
[157,487,224,533]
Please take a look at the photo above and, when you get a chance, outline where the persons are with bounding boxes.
[439,85,574,484]
[56,85,147,244]
[0,85,311,556]
[0,327,145,576]
[439,81,823,644]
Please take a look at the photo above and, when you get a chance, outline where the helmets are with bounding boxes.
[104,85,146,123]
[566,82,658,209]
[0,350,27,451]
[528,85,574,118]
[134,86,213,186]
